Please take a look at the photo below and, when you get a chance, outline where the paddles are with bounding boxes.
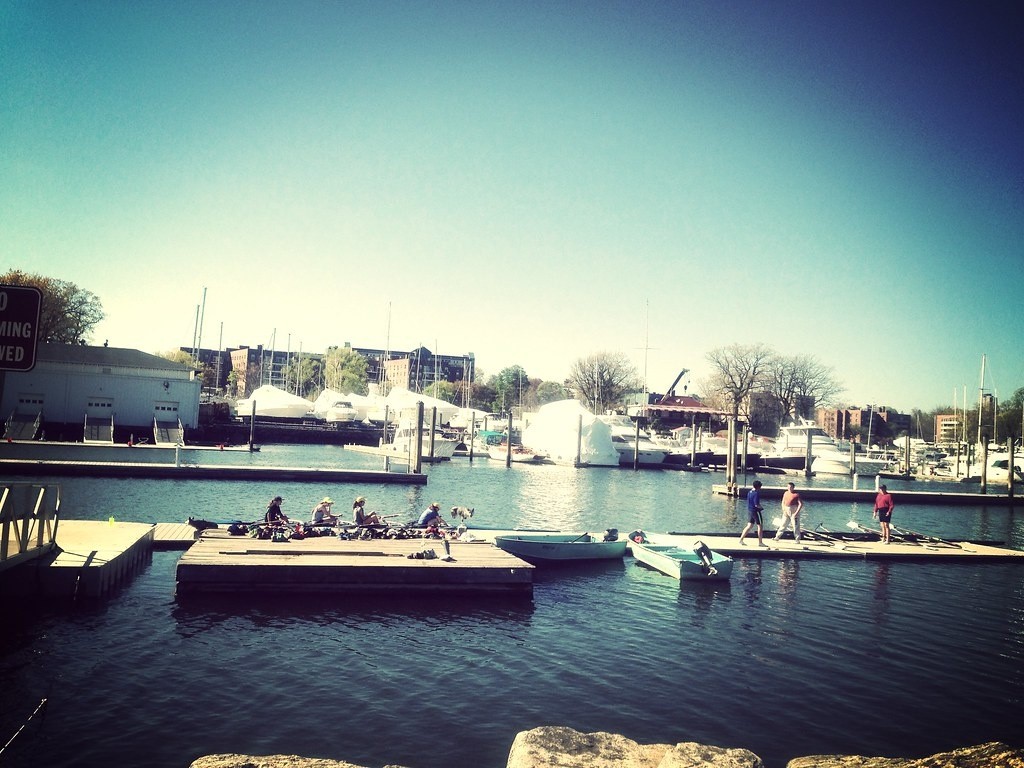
[858,525,905,541]
[894,525,963,549]
[803,528,840,541]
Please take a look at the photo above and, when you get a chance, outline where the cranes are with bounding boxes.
[661,365,691,403]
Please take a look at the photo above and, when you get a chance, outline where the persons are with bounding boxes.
[874,484,894,544]
[739,480,768,547]
[265,496,288,526]
[352,497,378,525]
[312,497,337,524]
[772,482,803,544]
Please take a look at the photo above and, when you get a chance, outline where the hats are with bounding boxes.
[879,484,886,489]
[321,497,334,504]
[273,496,284,501]
[356,497,367,502]
[433,503,441,507]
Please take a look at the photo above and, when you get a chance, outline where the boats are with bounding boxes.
[810,449,889,478]
[493,533,627,561]
[596,411,669,465]
[688,432,762,469]
[324,394,358,423]
[235,385,316,418]
[379,415,464,462]
[313,385,523,439]
[487,444,544,463]
[650,433,716,469]
[772,416,843,473]
[740,437,819,473]
[628,529,734,580]
[867,353,1024,483]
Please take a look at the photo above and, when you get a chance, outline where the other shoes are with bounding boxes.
[759,543,768,547]
[739,540,746,545]
[795,541,800,543]
[772,537,779,541]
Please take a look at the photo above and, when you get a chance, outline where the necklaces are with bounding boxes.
[418,503,447,526]
